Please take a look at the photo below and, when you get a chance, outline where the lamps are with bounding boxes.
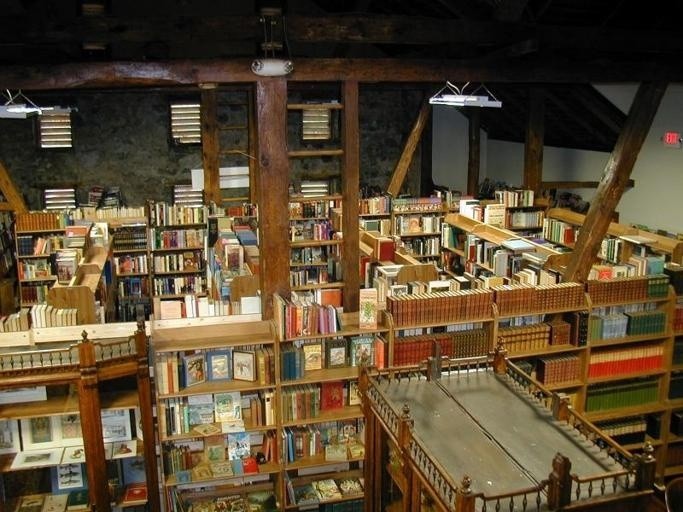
[251,8,294,76]
[428,82,502,108]
[0,88,56,121]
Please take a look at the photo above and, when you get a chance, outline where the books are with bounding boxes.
[0,190,683,512]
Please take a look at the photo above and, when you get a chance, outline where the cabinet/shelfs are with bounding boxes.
[3,197,683,512]
